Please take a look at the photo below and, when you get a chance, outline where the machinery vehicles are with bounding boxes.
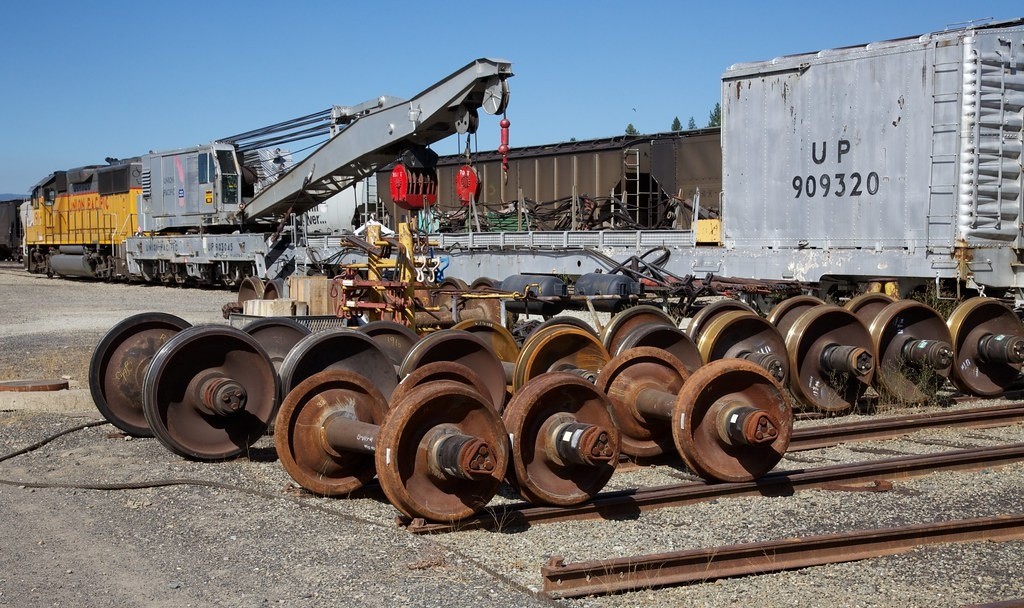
[20,57,516,282]
